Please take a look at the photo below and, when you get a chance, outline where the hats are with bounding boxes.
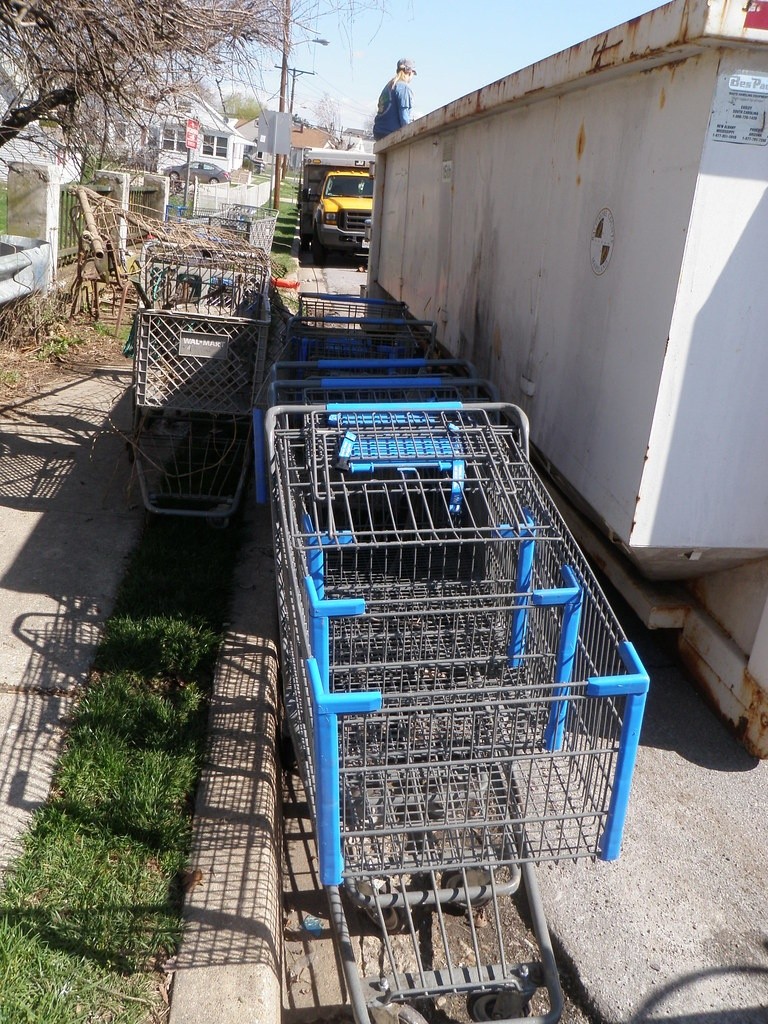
[396,57,418,76]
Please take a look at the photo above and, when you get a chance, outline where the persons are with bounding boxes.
[372,57,418,142]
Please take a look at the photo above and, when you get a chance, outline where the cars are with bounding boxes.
[164,161,231,184]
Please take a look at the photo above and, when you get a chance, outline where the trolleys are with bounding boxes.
[129,204,649,1024]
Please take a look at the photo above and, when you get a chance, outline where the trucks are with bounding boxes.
[303,148,376,266]
